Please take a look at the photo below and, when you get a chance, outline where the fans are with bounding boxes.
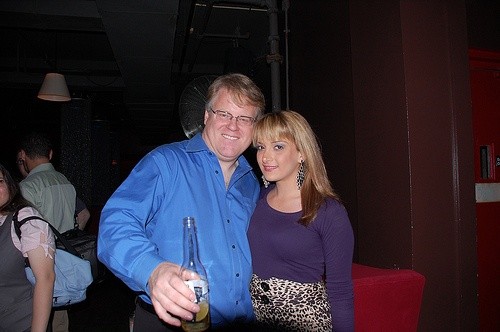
[178,75,220,139]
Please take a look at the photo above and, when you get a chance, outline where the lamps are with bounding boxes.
[36,31,72,102]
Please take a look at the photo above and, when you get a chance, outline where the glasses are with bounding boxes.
[210,107,255,126]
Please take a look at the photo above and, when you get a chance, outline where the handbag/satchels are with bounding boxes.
[23,227,107,309]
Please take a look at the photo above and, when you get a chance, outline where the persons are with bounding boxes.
[16,138,90,332]
[0,165,55,332]
[97,73,266,332]
[246,110,355,332]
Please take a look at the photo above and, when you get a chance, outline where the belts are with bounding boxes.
[135,297,156,316]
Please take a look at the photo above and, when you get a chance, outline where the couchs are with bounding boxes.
[351,260,426,332]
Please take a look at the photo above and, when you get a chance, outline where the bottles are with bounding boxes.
[179,217,210,331]
